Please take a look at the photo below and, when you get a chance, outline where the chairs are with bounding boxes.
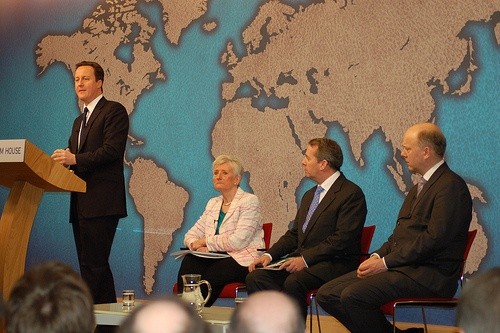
[306,225,376,333]
[380,229,478,333]
[173,223,273,298]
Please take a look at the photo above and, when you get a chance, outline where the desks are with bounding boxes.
[93,301,236,333]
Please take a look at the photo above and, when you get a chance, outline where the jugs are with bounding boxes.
[179,274,211,319]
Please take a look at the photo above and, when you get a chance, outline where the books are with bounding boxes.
[170,250,230,265]
[255,258,287,271]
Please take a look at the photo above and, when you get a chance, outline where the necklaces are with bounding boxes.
[224,200,233,205]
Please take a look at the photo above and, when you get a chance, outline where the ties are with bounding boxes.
[417,177,427,197]
[302,186,324,233]
[80,107,88,147]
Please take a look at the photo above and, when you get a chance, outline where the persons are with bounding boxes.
[176,156,270,308]
[50,60,127,305]
[244,138,367,325]
[118,294,210,333]
[229,289,306,333]
[317,123,472,333]
[6,261,98,333]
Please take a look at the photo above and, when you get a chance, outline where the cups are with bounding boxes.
[122,289,136,313]
[234,298,248,308]
